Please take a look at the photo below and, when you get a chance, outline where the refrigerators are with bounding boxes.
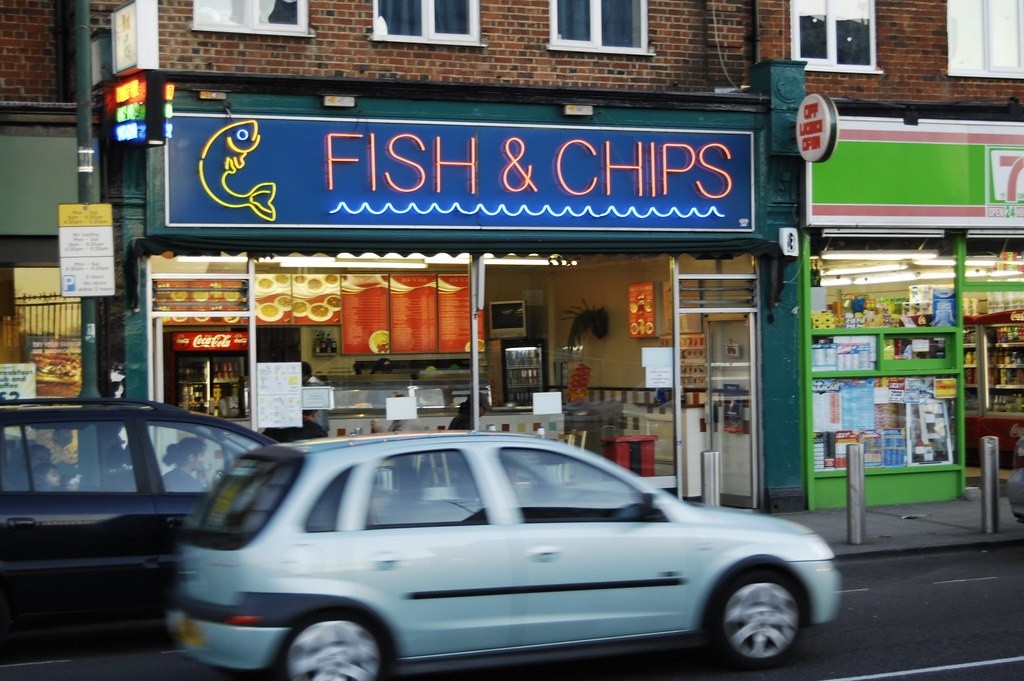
[164,332,251,420]
[502,338,546,406]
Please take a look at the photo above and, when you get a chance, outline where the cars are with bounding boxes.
[1,394,282,647]
[165,430,842,680]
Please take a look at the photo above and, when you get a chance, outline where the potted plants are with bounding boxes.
[560,296,608,350]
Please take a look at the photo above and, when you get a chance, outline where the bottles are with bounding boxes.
[506,351,540,405]
[179,361,234,417]
[964,326,1024,413]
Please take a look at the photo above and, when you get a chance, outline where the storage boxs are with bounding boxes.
[811,284,988,329]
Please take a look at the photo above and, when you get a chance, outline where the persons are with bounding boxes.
[370,358,395,374]
[904,344,931,447]
[301,361,329,432]
[162,437,213,491]
[13,444,62,490]
[448,394,492,431]
[261,410,329,444]
[367,470,425,527]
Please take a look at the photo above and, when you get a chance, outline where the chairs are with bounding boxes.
[106,442,138,492]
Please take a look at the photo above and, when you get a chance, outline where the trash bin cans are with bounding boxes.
[599,434,659,477]
[563,399,625,457]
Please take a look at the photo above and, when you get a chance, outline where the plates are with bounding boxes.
[171,292,240,323]
[368,330,389,354]
[256,274,341,322]
